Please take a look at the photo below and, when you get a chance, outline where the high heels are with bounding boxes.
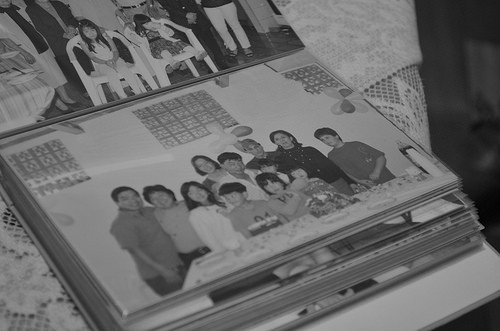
[60,100,84,109]
[244,48,255,59]
[229,48,239,57]
[56,104,74,115]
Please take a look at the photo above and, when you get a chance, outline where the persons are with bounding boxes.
[110,128,397,296]
[0,0,254,131]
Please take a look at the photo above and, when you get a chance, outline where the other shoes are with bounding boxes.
[195,50,209,62]
[171,62,182,72]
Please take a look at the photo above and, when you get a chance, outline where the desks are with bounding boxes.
[0,0,433,331]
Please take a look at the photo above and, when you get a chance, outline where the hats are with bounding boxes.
[241,139,259,151]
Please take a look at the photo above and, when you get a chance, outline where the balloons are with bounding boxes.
[324,86,369,115]
[206,120,253,154]
[40,195,73,226]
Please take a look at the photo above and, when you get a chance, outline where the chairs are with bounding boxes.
[124,17,219,88]
[66,30,159,107]
